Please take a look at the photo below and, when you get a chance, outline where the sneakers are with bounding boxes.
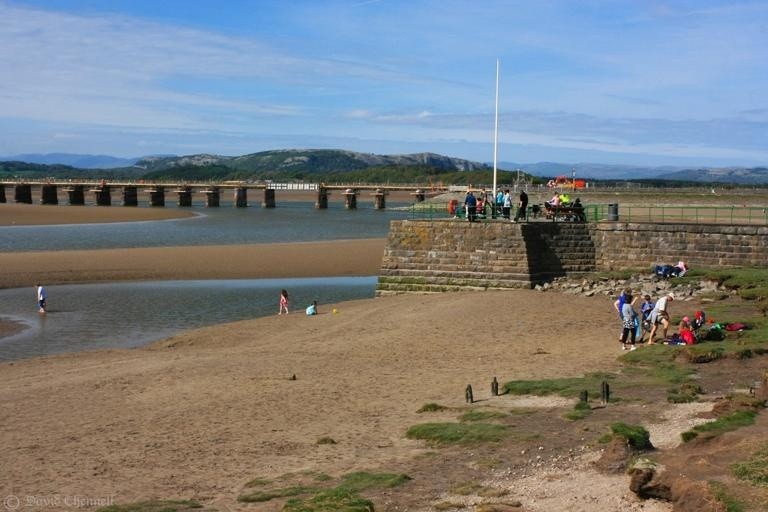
[622,347,637,351]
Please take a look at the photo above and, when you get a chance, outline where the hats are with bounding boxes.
[666,293,675,301]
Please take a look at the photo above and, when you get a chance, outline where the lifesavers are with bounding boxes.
[448,199,459,214]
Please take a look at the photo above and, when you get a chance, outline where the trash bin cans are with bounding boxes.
[449,200,458,214]
[607,203,618,221]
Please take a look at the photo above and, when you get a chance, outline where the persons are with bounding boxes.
[670,261,686,277]
[448,188,585,221]
[306,301,316,315]
[654,263,680,280]
[34,284,47,312]
[613,287,705,351]
[278,289,289,315]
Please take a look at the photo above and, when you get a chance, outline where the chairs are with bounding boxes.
[455,201,578,222]
